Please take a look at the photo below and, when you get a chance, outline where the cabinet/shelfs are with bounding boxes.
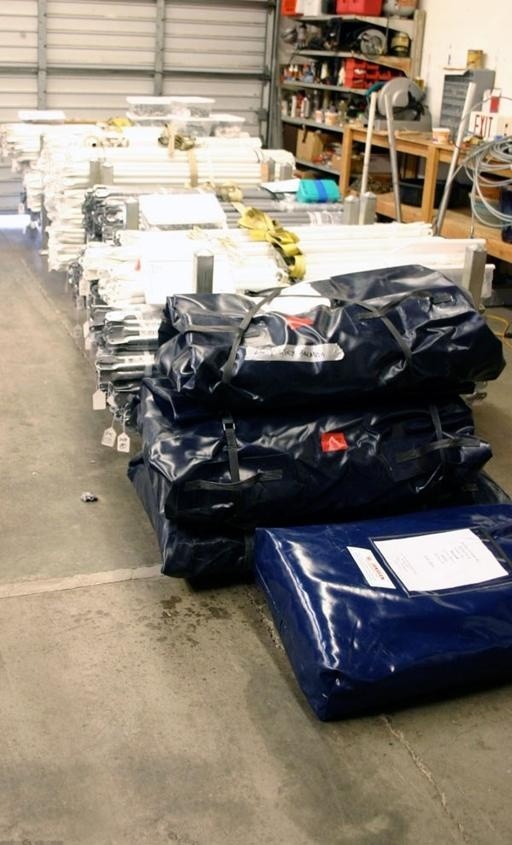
[280,9,425,193]
[440,68,495,134]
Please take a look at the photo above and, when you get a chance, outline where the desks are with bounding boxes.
[339,124,512,264]
